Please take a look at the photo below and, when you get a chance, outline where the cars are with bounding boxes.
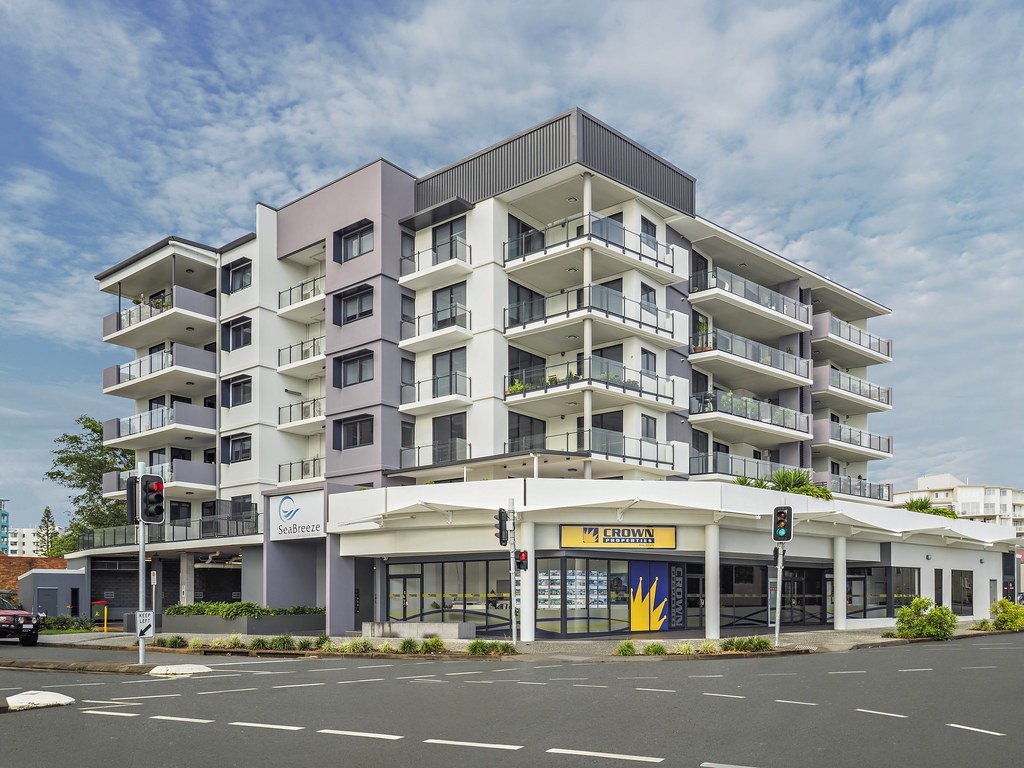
[0,598,40,647]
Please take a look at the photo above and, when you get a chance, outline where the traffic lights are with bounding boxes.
[125,473,167,524]
[514,549,530,572]
[493,508,508,547]
[770,505,793,542]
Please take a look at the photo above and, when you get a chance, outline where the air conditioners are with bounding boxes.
[302,343,320,359]
[303,286,320,300]
[302,400,320,419]
[303,458,319,479]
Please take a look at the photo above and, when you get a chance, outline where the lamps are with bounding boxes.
[568,467,578,473]
[568,401,577,405]
[186,381,194,385]
[186,268,194,274]
[185,436,192,441]
[568,268,577,272]
[567,335,577,339]
[814,350,820,354]
[814,300,821,304]
[926,554,931,560]
[567,195,577,203]
[186,326,195,331]
[185,492,194,495]
[980,559,985,563]
[813,452,821,454]
[738,263,747,268]
[814,401,820,403]
